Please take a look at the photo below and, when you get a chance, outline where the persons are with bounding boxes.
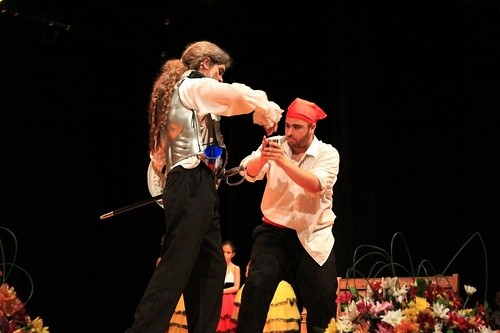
[212,241,241,332]
[130,40,285,333]
[230,259,302,333]
[154,234,188,333]
[235,98,340,333]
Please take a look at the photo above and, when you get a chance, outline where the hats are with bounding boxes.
[285,98,327,124]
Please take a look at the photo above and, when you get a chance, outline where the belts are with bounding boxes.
[262,216,291,229]
